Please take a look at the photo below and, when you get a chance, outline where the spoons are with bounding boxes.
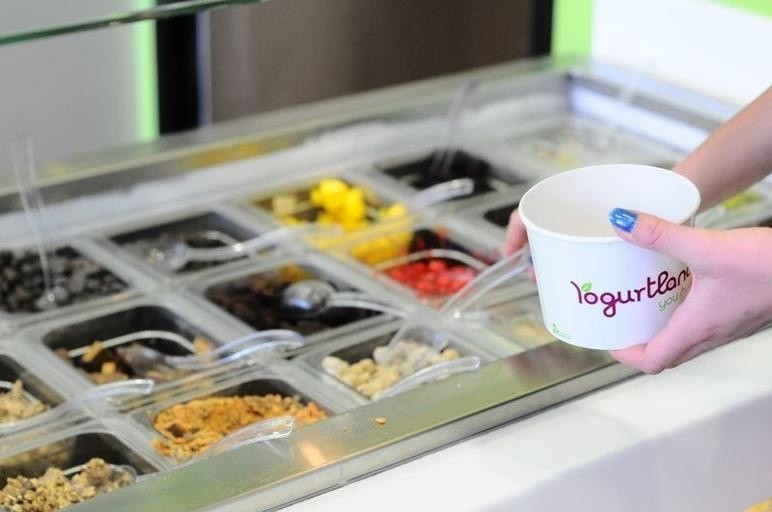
[279,278,413,319]
[376,242,532,377]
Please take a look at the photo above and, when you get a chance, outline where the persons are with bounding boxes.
[502,86,771,377]
